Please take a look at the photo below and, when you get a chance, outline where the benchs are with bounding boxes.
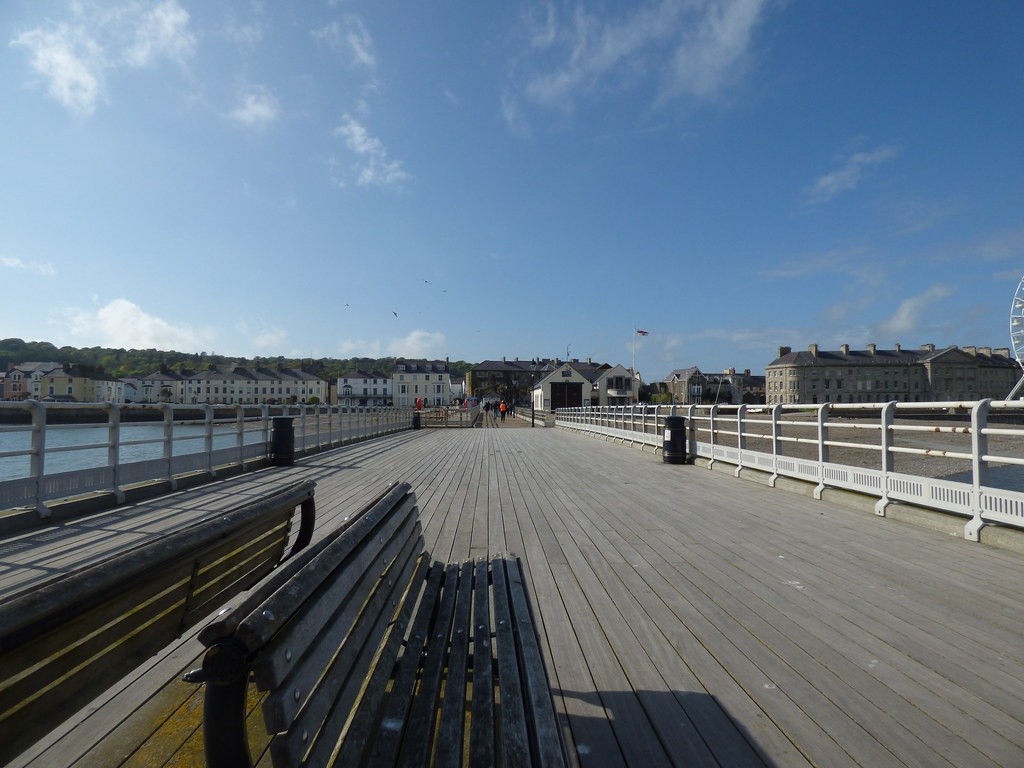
[198,479,569,768]
[0,477,318,768]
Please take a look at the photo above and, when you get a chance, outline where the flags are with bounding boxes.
[637,330,649,337]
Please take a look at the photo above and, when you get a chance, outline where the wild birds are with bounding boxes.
[420,279,432,286]
[392,311,399,317]
[441,290,447,293]
[344,303,350,306]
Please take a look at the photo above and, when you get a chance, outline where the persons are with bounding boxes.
[424,399,516,419]
[500,400,506,422]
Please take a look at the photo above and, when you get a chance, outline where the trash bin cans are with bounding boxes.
[271,418,294,467]
[413,412,421,429]
[663,415,687,464]
